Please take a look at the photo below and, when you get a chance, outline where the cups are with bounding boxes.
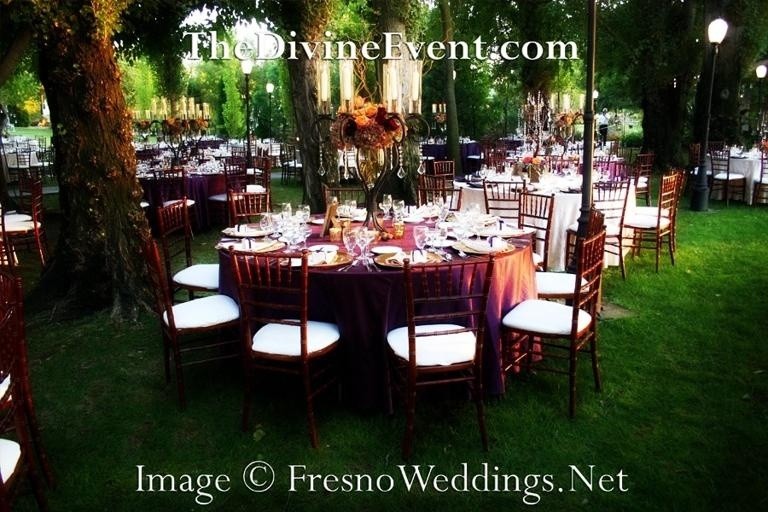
[134,154,236,177]
[469,162,611,195]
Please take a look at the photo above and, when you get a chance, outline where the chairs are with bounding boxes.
[683,130,766,208]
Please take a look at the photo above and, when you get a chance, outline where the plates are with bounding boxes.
[223,193,534,272]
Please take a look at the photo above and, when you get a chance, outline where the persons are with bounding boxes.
[597,106,611,146]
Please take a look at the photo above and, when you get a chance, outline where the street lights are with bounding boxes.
[240,58,256,169]
[469,63,476,137]
[594,90,600,142]
[754,64,767,140]
[265,82,275,155]
[693,19,729,213]
